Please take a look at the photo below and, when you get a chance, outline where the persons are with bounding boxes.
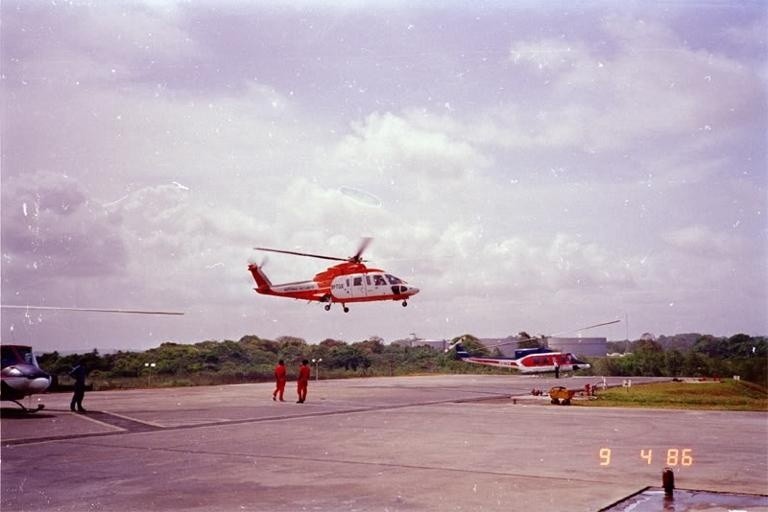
[295,358,311,402]
[69,357,88,412]
[272,359,286,402]
[554,360,560,378]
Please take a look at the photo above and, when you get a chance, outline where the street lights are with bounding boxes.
[312,358,323,382]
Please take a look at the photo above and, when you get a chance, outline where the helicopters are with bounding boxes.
[444,318,622,379]
[243,238,420,314]
[0,301,185,416]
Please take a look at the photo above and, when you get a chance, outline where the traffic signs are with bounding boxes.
[144,362,156,389]
